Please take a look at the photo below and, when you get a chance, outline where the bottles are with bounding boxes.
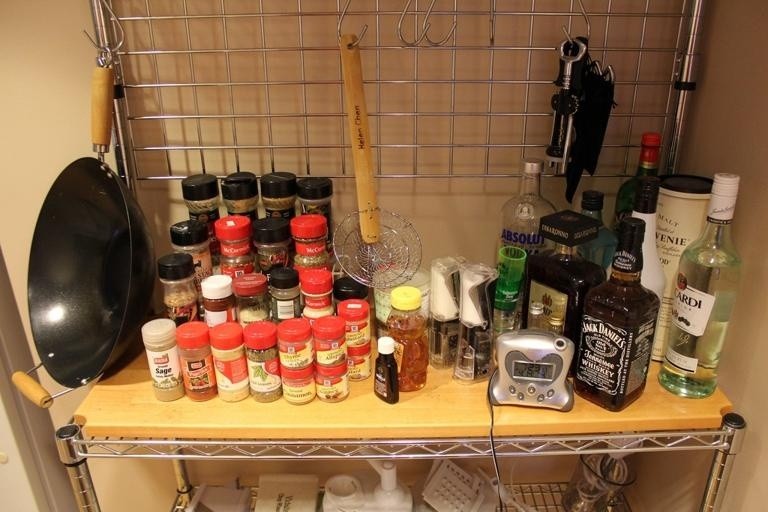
[257,172,297,221]
[276,318,316,406]
[169,221,212,317]
[141,318,184,400]
[295,268,335,322]
[157,254,200,323]
[242,321,282,405]
[574,191,616,265]
[500,157,553,255]
[494,246,527,333]
[231,273,269,326]
[607,132,660,233]
[207,322,249,403]
[571,218,662,411]
[373,461,405,509]
[332,277,369,315]
[265,268,300,322]
[338,298,373,382]
[217,172,260,223]
[248,217,289,271]
[657,172,742,398]
[632,175,666,300]
[430,255,499,389]
[176,322,218,403]
[310,316,349,404]
[294,176,334,250]
[524,208,603,377]
[200,274,235,327]
[651,171,714,362]
[287,212,332,271]
[181,173,222,266]
[373,336,399,406]
[213,214,254,276]
[385,285,429,393]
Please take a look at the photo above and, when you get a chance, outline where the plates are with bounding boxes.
[321,481,413,511]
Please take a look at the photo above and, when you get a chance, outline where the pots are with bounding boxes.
[10,46,156,410]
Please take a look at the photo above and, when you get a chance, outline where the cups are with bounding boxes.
[559,453,636,511]
[325,475,362,508]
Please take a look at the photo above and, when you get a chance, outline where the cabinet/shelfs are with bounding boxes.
[56,330,746,511]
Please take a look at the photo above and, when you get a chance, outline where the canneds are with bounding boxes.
[243,299,373,406]
